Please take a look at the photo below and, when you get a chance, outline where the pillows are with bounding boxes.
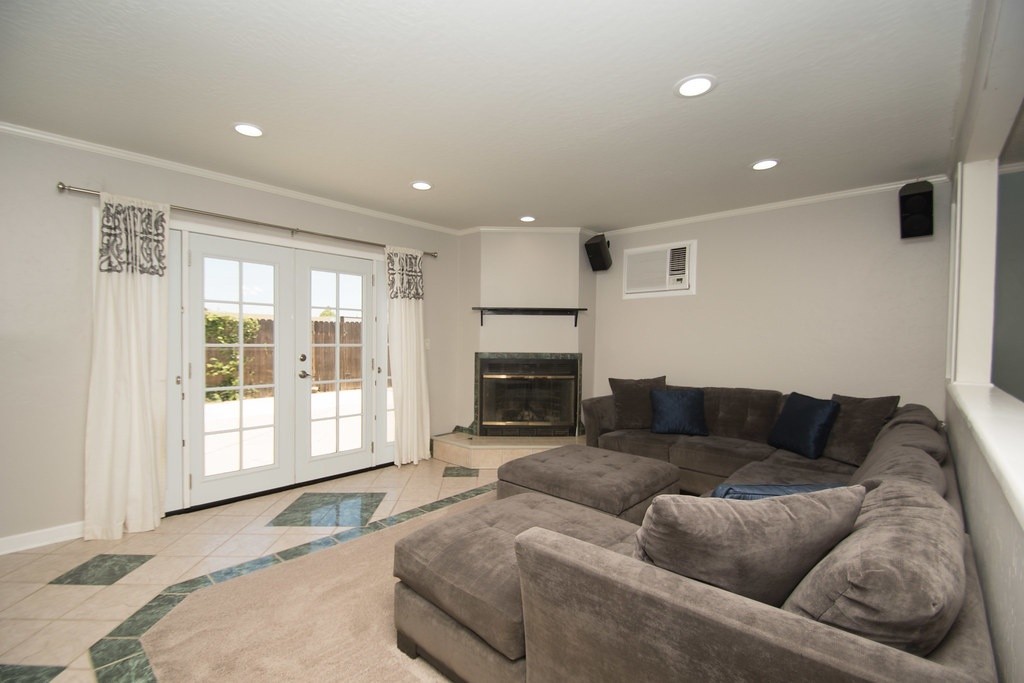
[609,375,666,430]
[771,391,840,459]
[630,485,866,605]
[825,393,899,466]
[646,388,709,436]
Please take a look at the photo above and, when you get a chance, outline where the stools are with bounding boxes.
[497,444,681,526]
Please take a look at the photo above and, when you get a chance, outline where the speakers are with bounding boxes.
[899,180,934,239]
[585,234,612,272]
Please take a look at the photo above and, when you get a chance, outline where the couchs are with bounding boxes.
[393,386,999,683]
